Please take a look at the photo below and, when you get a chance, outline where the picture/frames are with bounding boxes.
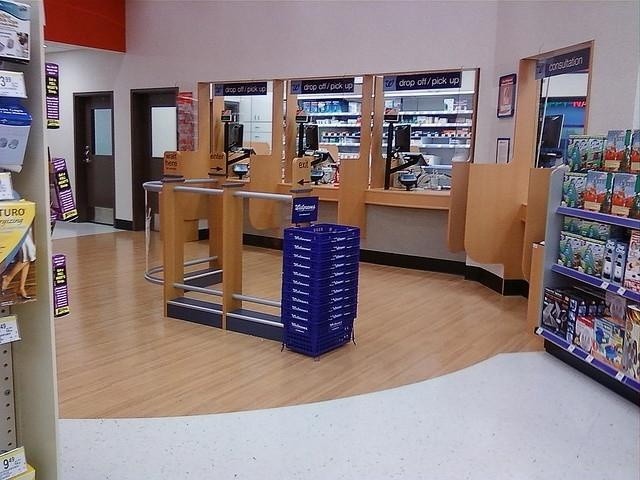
[497,74,516,117]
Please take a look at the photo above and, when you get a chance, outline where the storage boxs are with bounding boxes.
[280,223,360,358]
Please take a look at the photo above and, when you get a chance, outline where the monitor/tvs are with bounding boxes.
[395,125,410,152]
[306,125,318,150]
[538,114,565,149]
[230,123,244,148]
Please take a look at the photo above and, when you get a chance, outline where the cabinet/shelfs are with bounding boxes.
[0,1,61,479]
[535,161,640,408]
